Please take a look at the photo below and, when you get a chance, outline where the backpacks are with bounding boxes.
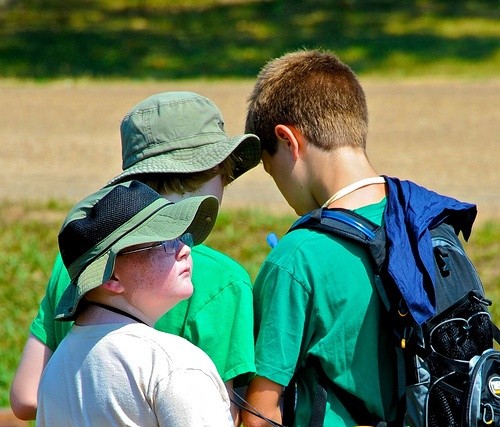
[284,175,500,427]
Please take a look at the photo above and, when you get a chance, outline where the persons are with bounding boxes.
[10,92,262,427]
[31,179,235,427]
[239,47,476,427]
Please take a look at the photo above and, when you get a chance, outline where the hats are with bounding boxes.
[102,91,261,185]
[53,180,220,321]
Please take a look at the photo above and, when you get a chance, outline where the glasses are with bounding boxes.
[116,231,194,255]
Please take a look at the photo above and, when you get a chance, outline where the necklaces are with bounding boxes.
[321,177,385,209]
[84,299,285,427]
